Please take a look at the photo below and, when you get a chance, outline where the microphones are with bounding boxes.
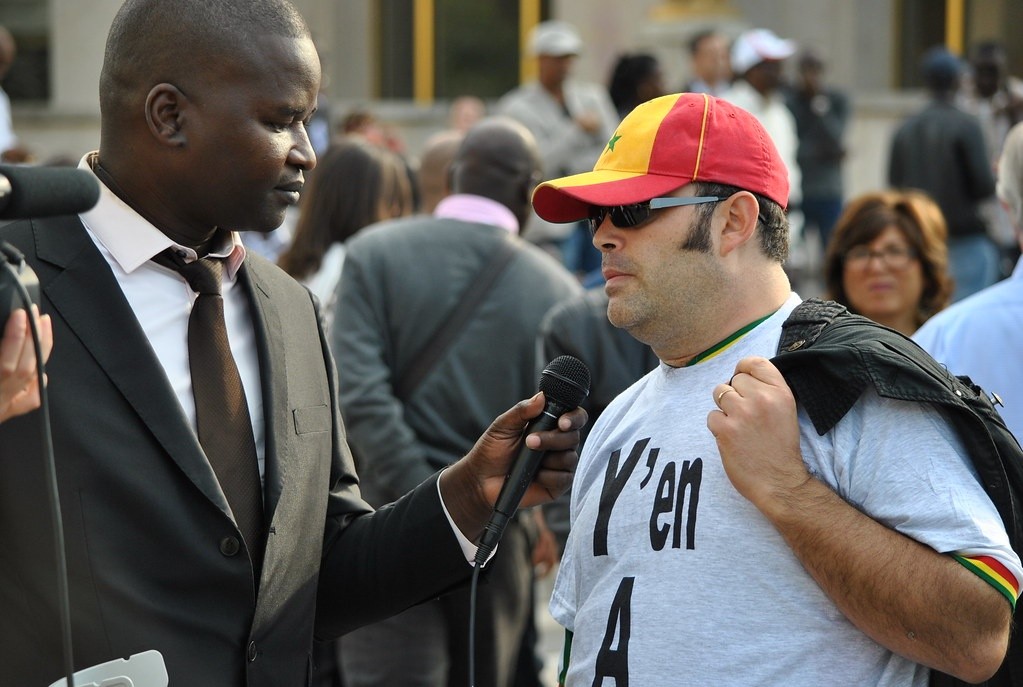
[474,355,592,568]
[0,167,100,221]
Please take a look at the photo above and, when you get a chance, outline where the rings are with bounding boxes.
[718,389,734,405]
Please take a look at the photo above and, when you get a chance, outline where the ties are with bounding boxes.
[153,247,269,580]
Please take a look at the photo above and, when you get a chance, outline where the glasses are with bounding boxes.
[842,243,921,272]
[588,196,768,237]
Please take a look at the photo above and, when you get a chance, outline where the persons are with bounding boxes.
[887,36,1023,302]
[240,96,485,313]
[0,0,590,687]
[532,94,1023,687]
[490,21,849,306]
[534,276,660,562]
[906,120,1023,450]
[333,117,591,685]
[819,185,951,337]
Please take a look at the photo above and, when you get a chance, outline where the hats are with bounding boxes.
[529,24,579,59]
[730,29,794,76]
[532,92,789,224]
